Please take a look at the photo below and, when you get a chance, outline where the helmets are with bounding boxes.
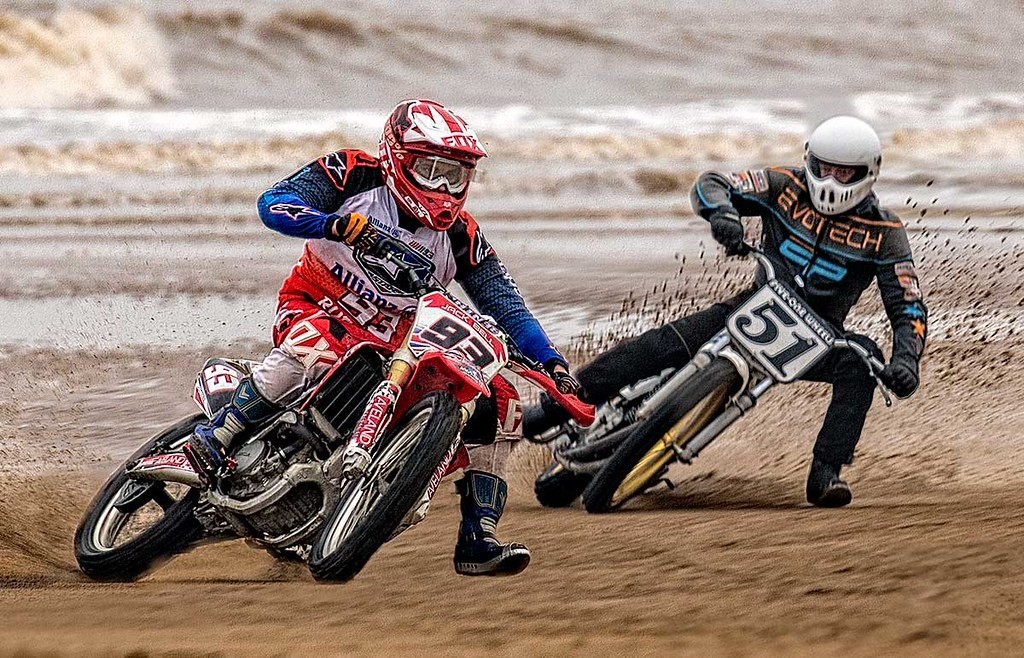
[804,116,882,214]
[379,99,488,231]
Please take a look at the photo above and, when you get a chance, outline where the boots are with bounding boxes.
[456,470,529,575]
[192,374,283,464]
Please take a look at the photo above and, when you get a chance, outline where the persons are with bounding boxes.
[516,116,928,517]
[195,99,588,577]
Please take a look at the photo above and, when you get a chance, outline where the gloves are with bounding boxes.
[884,364,918,398]
[337,214,379,248]
[710,206,744,256]
[542,372,588,410]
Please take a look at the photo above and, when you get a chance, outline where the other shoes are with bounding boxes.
[806,456,851,505]
[521,403,571,435]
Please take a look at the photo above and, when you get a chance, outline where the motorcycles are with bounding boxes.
[70,219,596,588]
[529,234,896,514]
